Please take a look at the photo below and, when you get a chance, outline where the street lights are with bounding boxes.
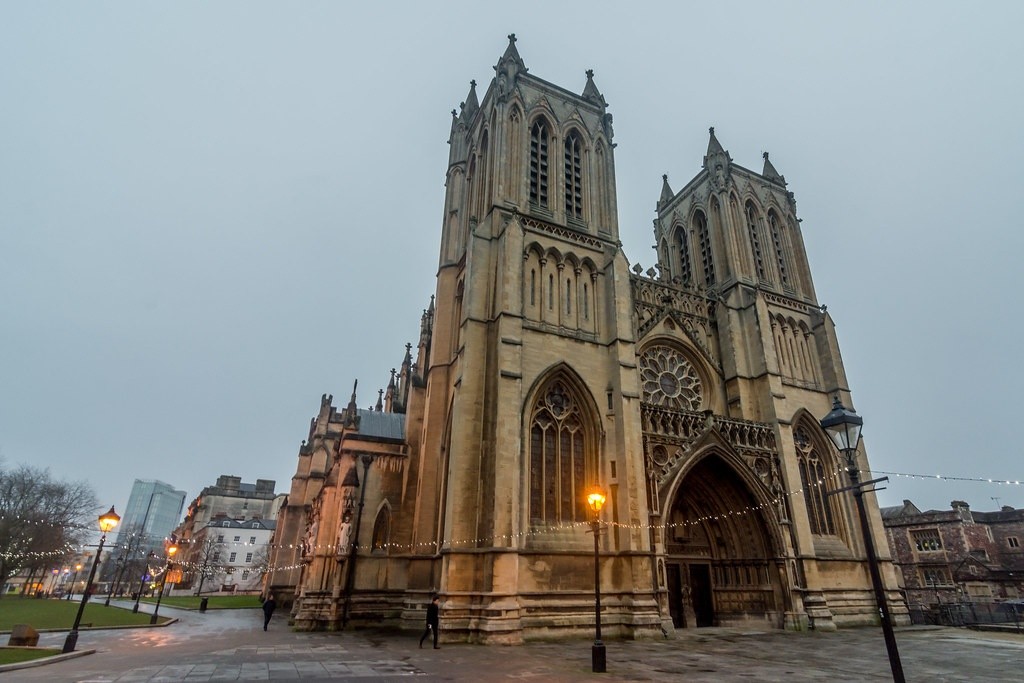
[131,549,156,613]
[822,396,906,683]
[47,568,69,599]
[149,542,176,625]
[104,556,124,608]
[584,480,608,673]
[67,564,81,601]
[65,504,121,652]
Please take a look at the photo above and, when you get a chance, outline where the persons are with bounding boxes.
[262,595,276,631]
[336,516,353,555]
[304,526,311,556]
[419,597,441,649]
[308,514,318,555]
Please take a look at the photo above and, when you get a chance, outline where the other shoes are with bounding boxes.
[263,627,267,631]
[418,645,423,649]
[434,646,440,649]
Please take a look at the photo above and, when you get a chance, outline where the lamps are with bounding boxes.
[753,283,760,294]
[469,216,478,231]
[816,305,827,313]
